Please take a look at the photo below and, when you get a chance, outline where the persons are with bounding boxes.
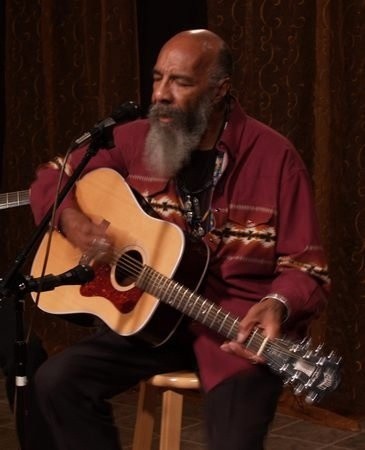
[17,29,331,450]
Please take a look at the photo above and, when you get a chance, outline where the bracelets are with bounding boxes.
[259,292,291,324]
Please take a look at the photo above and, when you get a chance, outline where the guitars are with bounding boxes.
[29,168,343,402]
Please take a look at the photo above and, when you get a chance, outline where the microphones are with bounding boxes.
[69,101,141,153]
[18,265,95,294]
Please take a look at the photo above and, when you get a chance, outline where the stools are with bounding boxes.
[133,369,201,450]
